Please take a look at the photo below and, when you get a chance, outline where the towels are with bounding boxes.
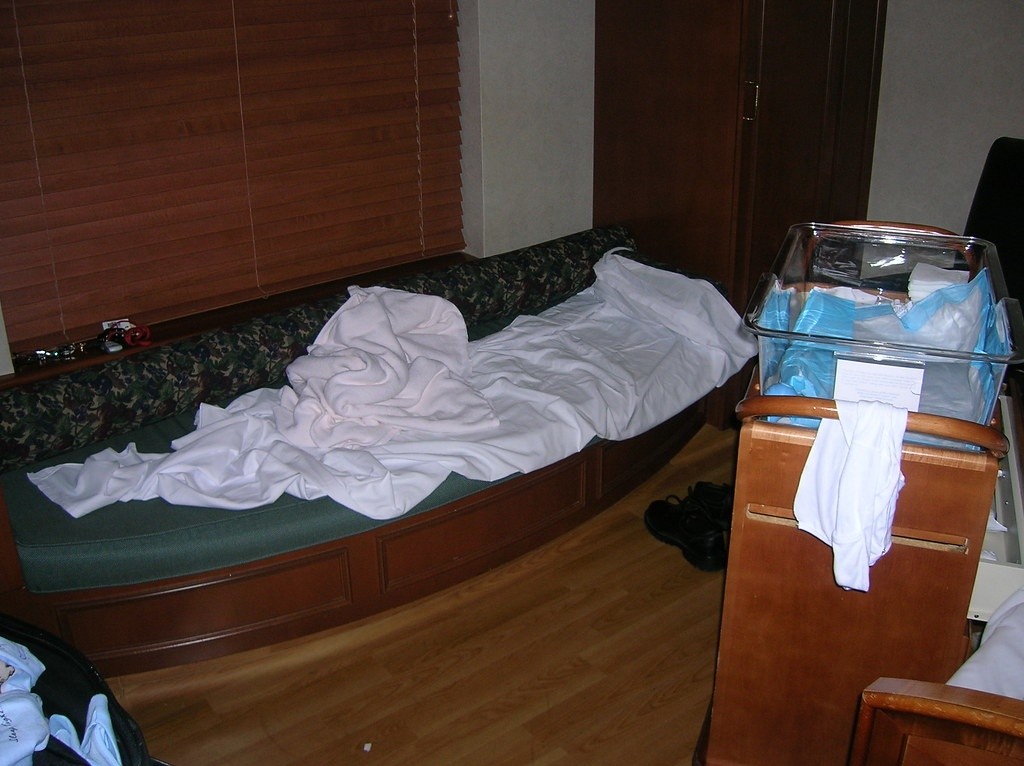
[792,398,910,594]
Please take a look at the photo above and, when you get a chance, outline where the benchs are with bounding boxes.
[0,222,709,680]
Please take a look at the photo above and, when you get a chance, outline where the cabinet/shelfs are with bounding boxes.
[595,0,885,431]
[702,227,1011,766]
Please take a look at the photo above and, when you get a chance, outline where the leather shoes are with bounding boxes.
[688,481,733,530]
[644,494,725,571]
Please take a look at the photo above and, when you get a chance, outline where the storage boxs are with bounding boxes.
[741,222,1024,453]
